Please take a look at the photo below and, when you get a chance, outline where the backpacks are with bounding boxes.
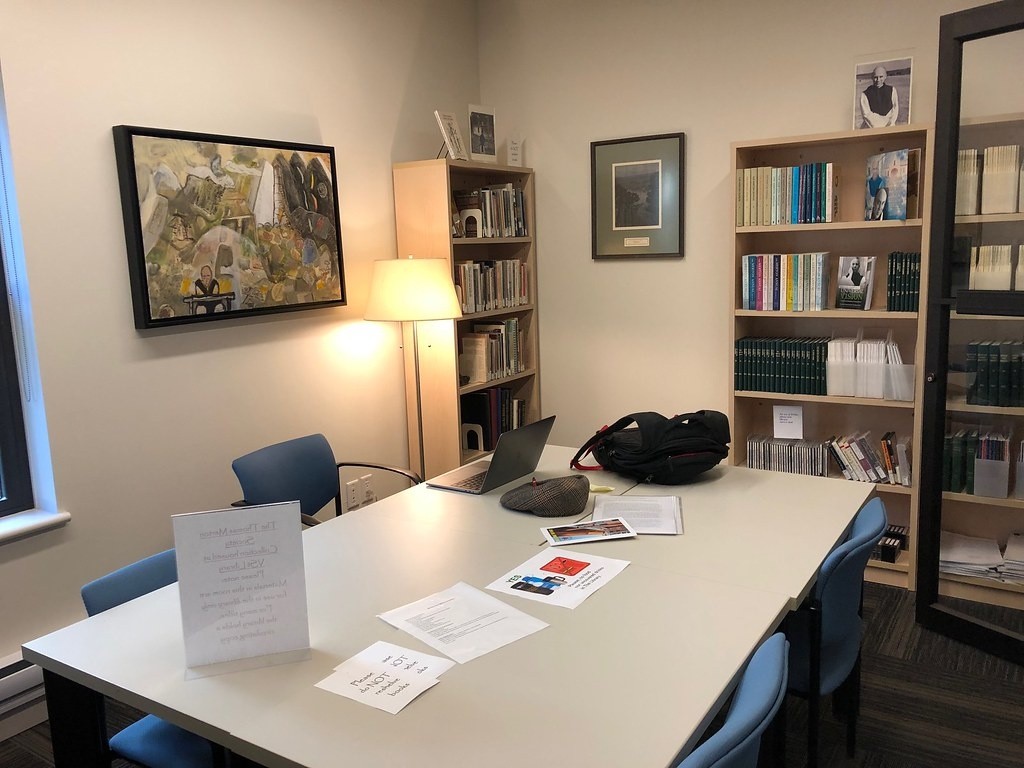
[569,410,733,486]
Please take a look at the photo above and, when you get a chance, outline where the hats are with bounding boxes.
[500,474,591,517]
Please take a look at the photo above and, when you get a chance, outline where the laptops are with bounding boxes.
[427,415,556,495]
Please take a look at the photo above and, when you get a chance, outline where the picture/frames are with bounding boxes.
[590,132,683,259]
[112,124,347,330]
[468,104,497,164]
[434,110,470,162]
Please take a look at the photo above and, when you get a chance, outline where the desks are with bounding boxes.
[0,445,877,768]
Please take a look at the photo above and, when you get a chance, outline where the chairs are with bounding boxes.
[676,632,790,768]
[82,546,178,617]
[107,714,256,768]
[775,497,889,768]
[230,433,423,527]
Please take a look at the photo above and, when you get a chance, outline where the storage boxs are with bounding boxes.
[856,361,883,400]
[884,363,915,403]
[974,452,1009,499]
[1016,455,1024,500]
[825,358,855,398]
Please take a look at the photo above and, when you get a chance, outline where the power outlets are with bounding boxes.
[346,479,360,510]
[360,473,376,504]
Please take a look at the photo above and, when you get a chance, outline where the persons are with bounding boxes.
[866,189,888,221]
[861,65,899,129]
[868,160,885,197]
[846,257,863,286]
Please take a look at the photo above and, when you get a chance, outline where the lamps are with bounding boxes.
[362,255,464,483]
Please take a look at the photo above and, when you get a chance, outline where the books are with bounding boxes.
[732,146,1024,588]
[447,168,532,460]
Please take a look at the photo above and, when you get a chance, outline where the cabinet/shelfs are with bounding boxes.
[392,158,541,489]
[727,110,1024,611]
